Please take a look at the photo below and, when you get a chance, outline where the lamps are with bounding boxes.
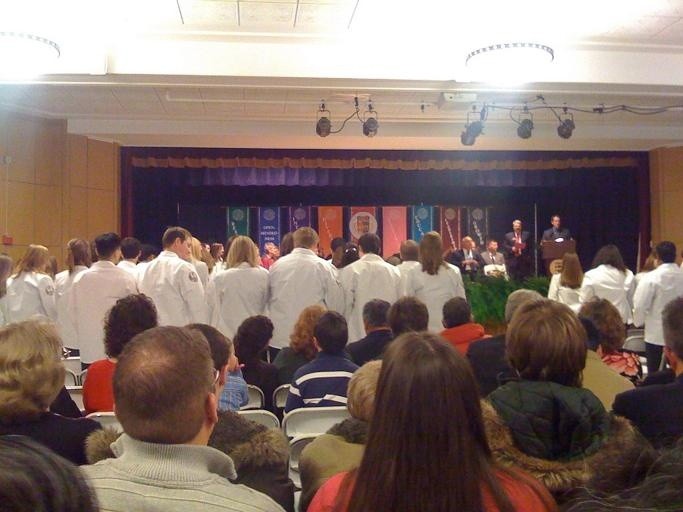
[316,105,331,138]
[516,103,534,138]
[557,106,575,138]
[362,103,378,137]
[2,31,61,80]
[464,42,555,86]
[460,105,484,145]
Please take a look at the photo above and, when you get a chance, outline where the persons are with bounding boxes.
[326,236,345,264]
[82,294,161,413]
[211,236,270,339]
[0,253,16,319]
[542,215,571,241]
[56,238,91,300]
[116,236,141,274]
[218,335,249,412]
[138,245,159,286]
[212,244,225,275]
[611,295,682,450]
[0,322,103,466]
[634,239,683,369]
[482,299,655,499]
[285,311,358,413]
[547,254,583,312]
[142,228,208,327]
[481,240,508,281]
[279,232,298,254]
[299,359,384,512]
[502,219,531,277]
[394,238,423,283]
[347,300,391,358]
[7,245,57,322]
[72,233,137,368]
[234,314,286,415]
[579,244,629,338]
[272,304,326,383]
[405,230,467,333]
[338,234,404,342]
[437,296,486,352]
[466,289,545,400]
[269,226,345,360]
[261,243,279,269]
[306,333,556,512]
[448,236,481,280]
[576,297,642,409]
[189,237,208,289]
[636,251,659,290]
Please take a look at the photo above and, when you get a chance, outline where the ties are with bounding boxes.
[516,232,519,252]
[467,249,469,262]
[491,255,494,263]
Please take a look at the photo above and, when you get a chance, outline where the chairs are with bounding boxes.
[62,357,84,370]
[63,369,78,383]
[234,410,280,433]
[79,370,88,384]
[84,412,123,435]
[65,384,86,414]
[240,386,266,409]
[273,384,293,409]
[622,334,648,376]
[288,433,322,506]
[282,406,352,437]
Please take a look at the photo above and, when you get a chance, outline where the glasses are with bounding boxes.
[211,368,218,394]
[61,347,70,362]
